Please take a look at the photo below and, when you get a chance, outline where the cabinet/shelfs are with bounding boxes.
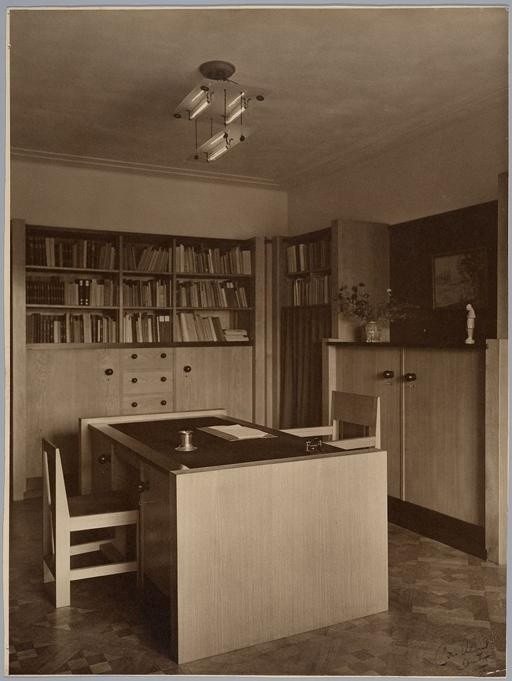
[284,226,330,308]
[335,346,485,549]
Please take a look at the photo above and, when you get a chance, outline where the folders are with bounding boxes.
[79,279,91,306]
[160,316,171,344]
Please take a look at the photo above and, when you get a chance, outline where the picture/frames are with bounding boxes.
[431,247,492,313]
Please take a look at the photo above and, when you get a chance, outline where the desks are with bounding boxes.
[77,409,389,664]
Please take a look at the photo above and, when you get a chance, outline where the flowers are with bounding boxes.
[335,279,421,322]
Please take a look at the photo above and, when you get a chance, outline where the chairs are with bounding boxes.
[279,390,381,451]
[40,437,140,609]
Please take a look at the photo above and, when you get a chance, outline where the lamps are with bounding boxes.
[173,61,254,165]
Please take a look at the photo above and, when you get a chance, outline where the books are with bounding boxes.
[285,239,331,307]
[25,234,253,344]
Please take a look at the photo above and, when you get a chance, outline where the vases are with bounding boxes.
[360,320,383,343]
